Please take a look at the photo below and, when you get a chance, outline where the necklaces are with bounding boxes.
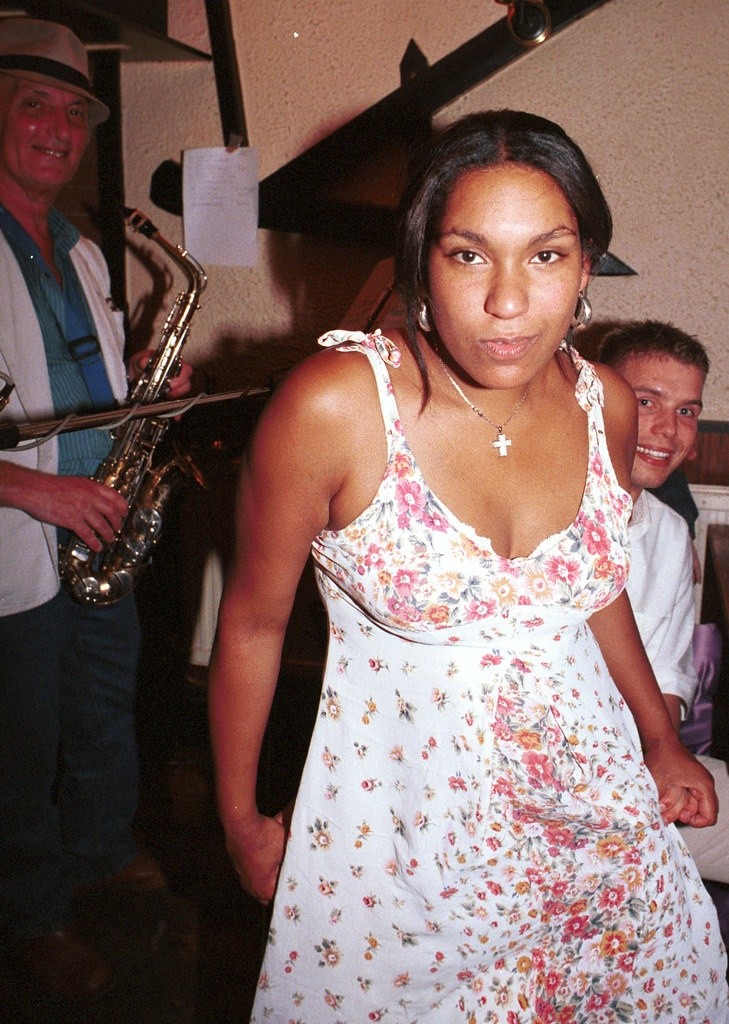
[421,325,545,459]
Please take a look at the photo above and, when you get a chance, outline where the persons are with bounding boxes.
[201,108,726,1024]
[0,19,192,992]
[590,324,722,828]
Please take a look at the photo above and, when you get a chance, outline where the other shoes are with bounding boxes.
[21,918,116,1003]
[98,859,165,895]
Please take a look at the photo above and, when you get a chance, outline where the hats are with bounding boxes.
[4,19,108,124]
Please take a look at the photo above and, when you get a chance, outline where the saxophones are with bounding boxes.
[55,204,214,603]
